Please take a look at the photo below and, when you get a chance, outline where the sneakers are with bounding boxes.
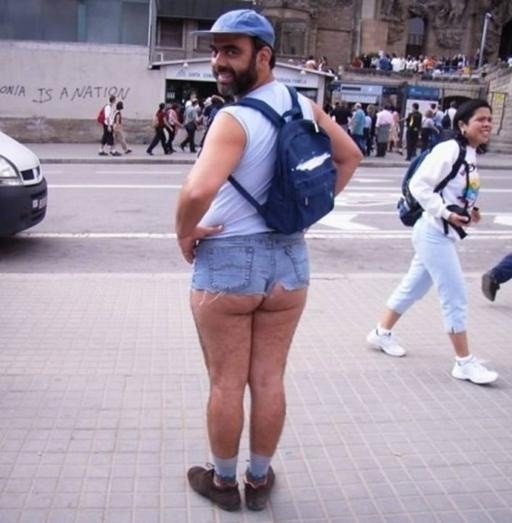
[451,354,498,385]
[366,327,407,357]
[187,466,241,512]
[243,461,275,512]
[98,142,197,157]
[480,271,499,301]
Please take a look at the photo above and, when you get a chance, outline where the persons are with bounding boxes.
[321,101,459,162]
[147,103,179,155]
[480,250,511,304]
[112,101,133,155]
[297,47,511,84]
[178,89,224,154]
[98,94,122,155]
[173,8,365,515]
[362,95,502,389]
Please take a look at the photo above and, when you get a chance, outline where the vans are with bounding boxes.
[0,127,47,246]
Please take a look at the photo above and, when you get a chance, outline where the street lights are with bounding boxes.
[477,11,492,67]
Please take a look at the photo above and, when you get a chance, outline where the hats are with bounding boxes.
[189,9,276,49]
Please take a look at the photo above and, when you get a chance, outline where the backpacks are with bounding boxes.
[198,84,337,237]
[396,130,467,227]
[97,103,108,124]
